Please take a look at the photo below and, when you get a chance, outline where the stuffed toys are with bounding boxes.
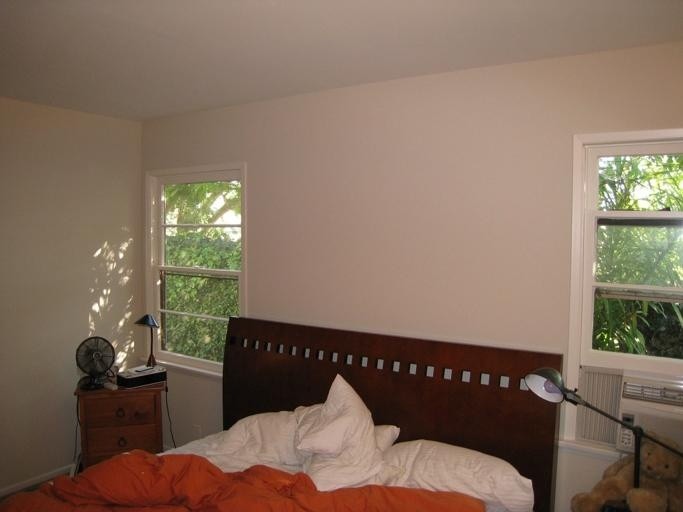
[570,430,683,512]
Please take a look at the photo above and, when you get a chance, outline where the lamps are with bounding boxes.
[133,313,160,366]
[523,369,682,510]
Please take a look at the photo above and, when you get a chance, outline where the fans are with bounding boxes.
[76,336,115,392]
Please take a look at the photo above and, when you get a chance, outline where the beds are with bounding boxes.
[0,314,563,511]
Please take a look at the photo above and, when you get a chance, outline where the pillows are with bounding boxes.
[212,374,534,512]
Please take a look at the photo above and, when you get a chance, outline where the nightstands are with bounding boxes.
[73,375,167,471]
